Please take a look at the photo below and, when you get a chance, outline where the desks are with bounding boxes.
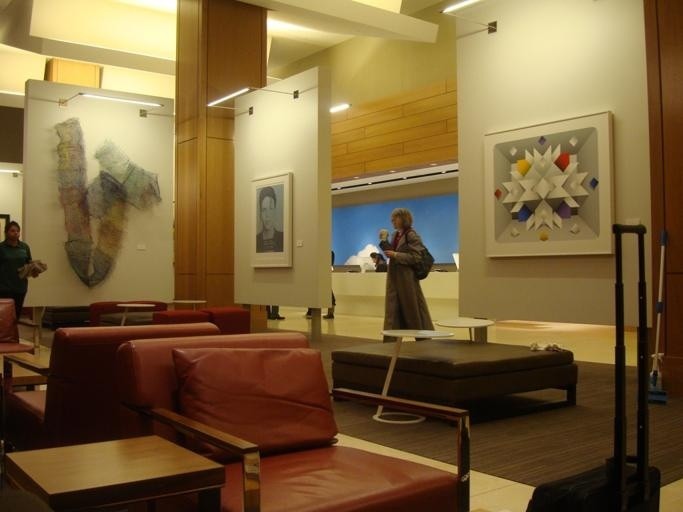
[373,330,454,426]
[435,316,494,342]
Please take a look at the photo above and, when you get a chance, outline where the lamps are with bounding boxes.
[59,92,164,116]
[206,86,298,115]
[438,0,497,34]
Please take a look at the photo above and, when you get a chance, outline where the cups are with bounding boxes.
[379,229,389,241]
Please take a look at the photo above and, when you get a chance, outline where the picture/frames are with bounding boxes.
[483,111,615,259]
[249,172,293,268]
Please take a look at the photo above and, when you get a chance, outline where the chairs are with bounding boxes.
[114,332,470,512]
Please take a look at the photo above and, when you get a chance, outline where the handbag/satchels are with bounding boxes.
[412,247,434,279]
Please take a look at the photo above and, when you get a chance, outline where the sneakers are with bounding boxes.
[323,314,334,318]
[307,311,311,317]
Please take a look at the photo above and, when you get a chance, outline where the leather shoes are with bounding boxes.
[273,314,284,319]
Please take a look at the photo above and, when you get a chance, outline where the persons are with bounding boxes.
[381,204,435,344]
[266,305,285,320]
[369,251,388,275]
[0,221,39,323]
[255,187,283,253]
[306,251,335,320]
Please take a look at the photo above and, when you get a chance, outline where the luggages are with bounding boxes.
[527,224,660,511]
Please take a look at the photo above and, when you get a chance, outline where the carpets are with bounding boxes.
[266,328,683,488]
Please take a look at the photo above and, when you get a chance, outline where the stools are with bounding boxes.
[333,339,577,412]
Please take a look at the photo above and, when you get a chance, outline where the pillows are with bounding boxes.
[173,348,337,462]
